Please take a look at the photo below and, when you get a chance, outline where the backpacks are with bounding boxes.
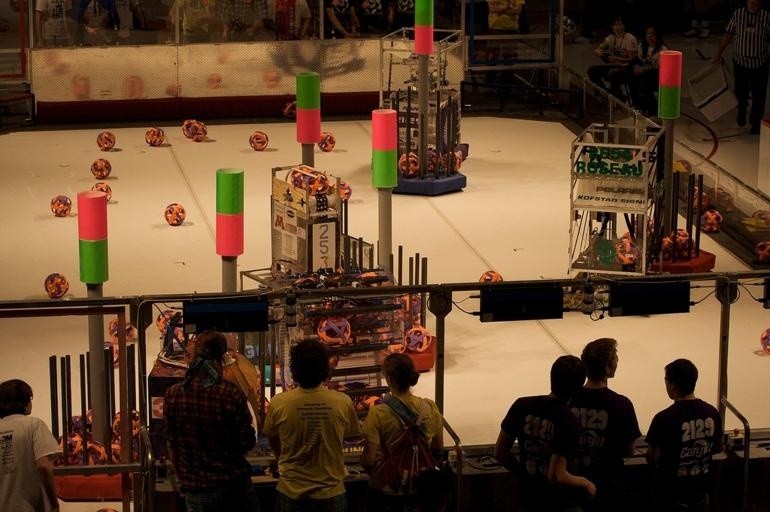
[374,399,435,494]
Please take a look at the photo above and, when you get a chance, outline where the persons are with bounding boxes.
[264,340,360,511]
[645,357,723,511]
[33,1,414,46]
[165,331,257,511]
[548,339,644,512]
[708,0,770,134]
[487,1,525,58]
[495,355,585,510]
[587,19,637,107]
[0,380,64,511]
[366,353,446,511]
[630,25,666,119]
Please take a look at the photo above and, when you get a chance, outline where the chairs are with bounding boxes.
[610,42,643,107]
